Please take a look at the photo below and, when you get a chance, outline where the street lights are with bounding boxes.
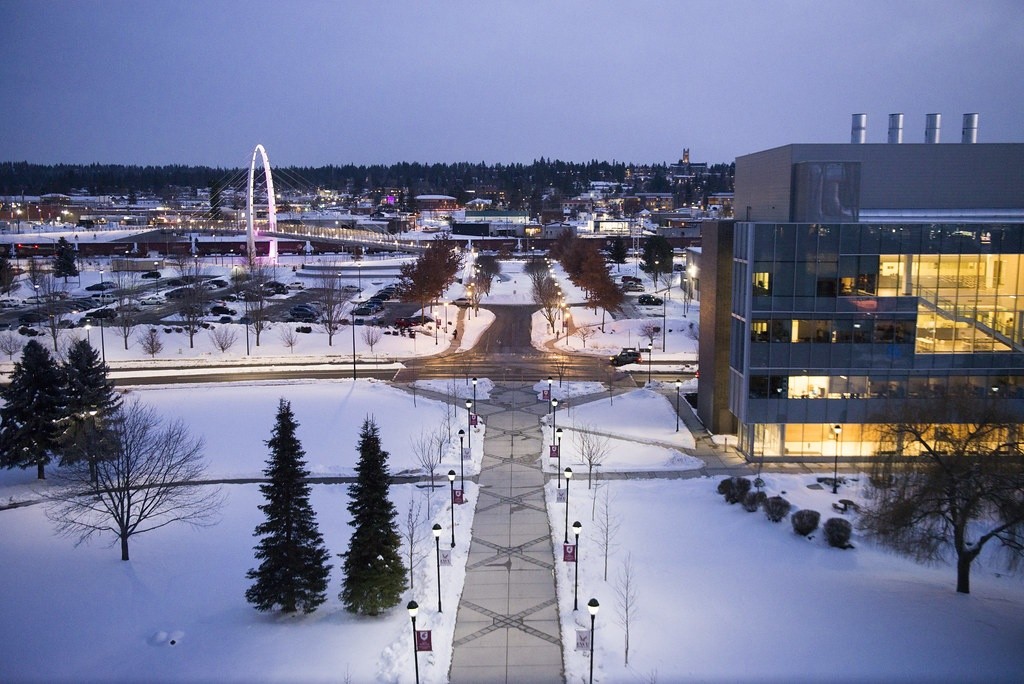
[466,399,473,448]
[648,343,652,384]
[552,398,558,446]
[458,429,466,494]
[675,379,682,432]
[407,600,419,684]
[556,428,563,488]
[471,377,477,428]
[564,467,573,544]
[432,523,443,613]
[548,376,553,414]
[833,424,841,494]
[572,521,583,611]
[587,598,599,684]
[447,470,457,548]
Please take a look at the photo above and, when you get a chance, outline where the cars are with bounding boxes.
[350,287,396,316]
[638,294,663,306]
[621,275,645,292]
[609,351,643,367]
[289,301,333,319]
